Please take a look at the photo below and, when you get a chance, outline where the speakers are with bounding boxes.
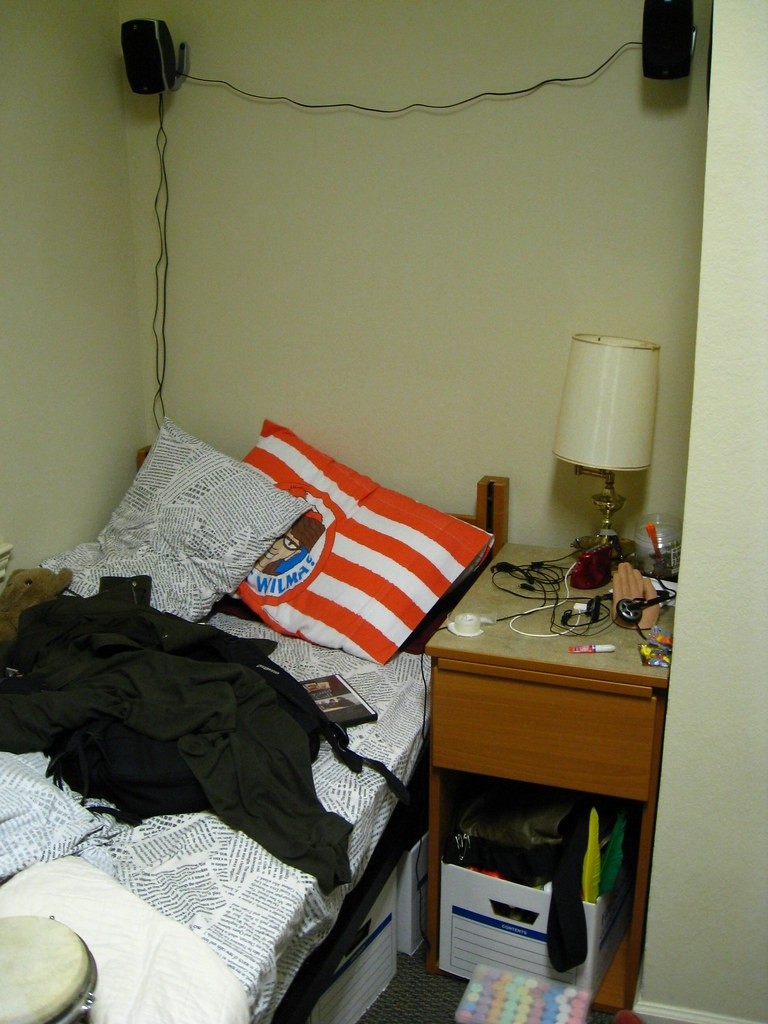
[642,0,696,81]
[121,18,187,94]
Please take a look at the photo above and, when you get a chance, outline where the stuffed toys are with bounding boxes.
[0,566,72,643]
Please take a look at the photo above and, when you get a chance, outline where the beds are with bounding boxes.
[0,416,510,1024]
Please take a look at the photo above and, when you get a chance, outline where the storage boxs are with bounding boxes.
[391,830,430,955]
[306,866,398,1024]
[438,843,635,1005]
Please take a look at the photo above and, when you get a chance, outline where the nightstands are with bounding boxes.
[427,541,681,1010]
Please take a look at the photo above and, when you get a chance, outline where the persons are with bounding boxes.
[611,563,660,630]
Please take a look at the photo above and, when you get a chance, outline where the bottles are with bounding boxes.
[635,513,682,580]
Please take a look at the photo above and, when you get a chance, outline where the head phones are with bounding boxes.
[583,587,671,624]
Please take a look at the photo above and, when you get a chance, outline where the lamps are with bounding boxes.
[552,333,660,592]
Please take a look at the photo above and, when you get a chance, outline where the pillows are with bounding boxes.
[0,855,250,1024]
[229,418,494,666]
[63,447,314,623]
[35,416,278,582]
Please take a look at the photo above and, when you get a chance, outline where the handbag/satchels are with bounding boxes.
[442,781,618,971]
[45,631,411,829]
